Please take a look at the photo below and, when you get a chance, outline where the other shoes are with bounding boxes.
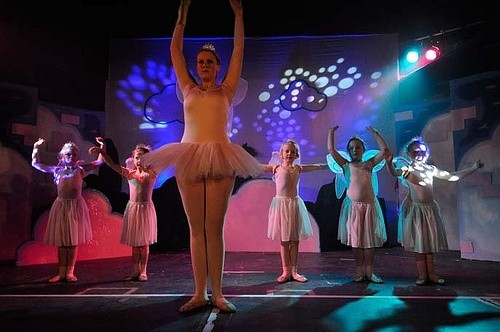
[430,278,445,284]
[49,276,66,282]
[277,274,291,282]
[352,274,366,281]
[366,274,384,284]
[123,276,138,281]
[416,279,425,284]
[291,274,307,281]
[139,275,148,281]
[65,276,78,282]
[210,300,236,312]
[179,301,207,312]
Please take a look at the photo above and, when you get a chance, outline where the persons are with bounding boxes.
[382,135,485,286]
[30,136,104,283]
[170,4,247,311]
[329,123,388,286]
[88,143,163,283]
[259,140,329,283]
